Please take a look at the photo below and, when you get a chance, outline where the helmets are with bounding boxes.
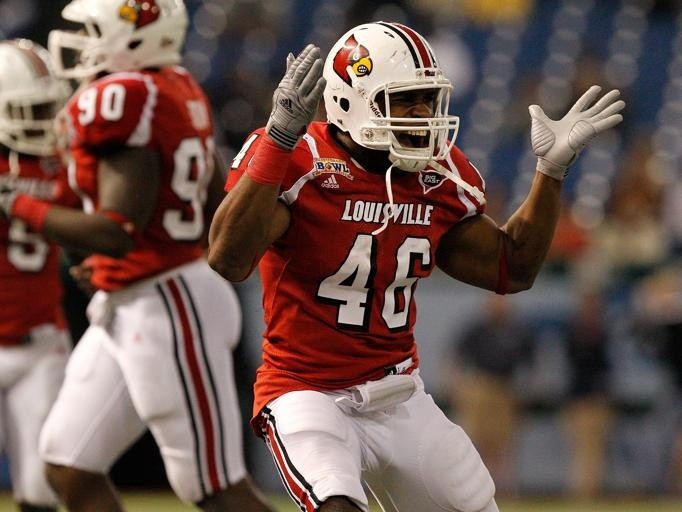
[0,38,73,156]
[49,0,189,80]
[321,21,461,174]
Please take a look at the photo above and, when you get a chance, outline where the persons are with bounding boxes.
[208,16,625,512]
[0,2,262,510]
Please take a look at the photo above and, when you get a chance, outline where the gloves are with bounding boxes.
[528,84,626,182]
[265,43,326,150]
